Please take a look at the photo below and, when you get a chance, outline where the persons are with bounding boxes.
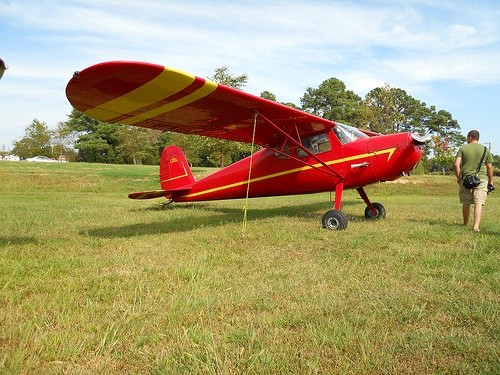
[454,130,496,232]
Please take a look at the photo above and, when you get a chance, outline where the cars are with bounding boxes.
[26,155,59,164]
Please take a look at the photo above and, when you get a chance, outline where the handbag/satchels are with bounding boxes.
[463,174,481,189]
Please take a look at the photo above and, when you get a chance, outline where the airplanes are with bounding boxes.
[66,60,434,231]
